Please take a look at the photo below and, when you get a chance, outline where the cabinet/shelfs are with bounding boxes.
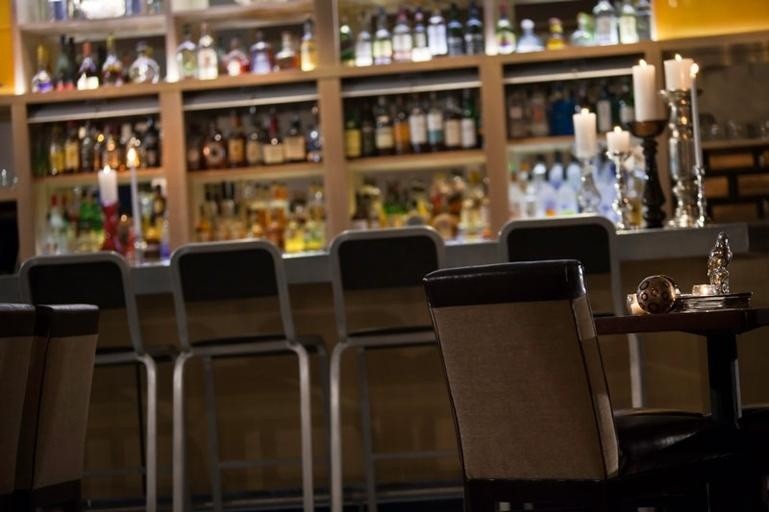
[0,0,769,267]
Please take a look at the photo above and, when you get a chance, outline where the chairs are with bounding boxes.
[420,258,707,512]
[1,299,102,512]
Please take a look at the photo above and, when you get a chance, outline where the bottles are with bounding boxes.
[507,75,635,142]
[352,166,489,239]
[342,89,484,158]
[47,115,162,175]
[175,12,320,81]
[24,1,165,19]
[509,144,621,223]
[495,0,653,54]
[41,184,170,263]
[184,102,323,171]
[339,1,486,68]
[193,178,329,252]
[30,34,161,95]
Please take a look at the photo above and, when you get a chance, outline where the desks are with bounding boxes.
[595,305,769,512]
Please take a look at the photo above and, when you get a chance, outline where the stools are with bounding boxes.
[330,227,449,511]
[17,249,181,512]
[171,237,331,511]
[498,215,643,407]
